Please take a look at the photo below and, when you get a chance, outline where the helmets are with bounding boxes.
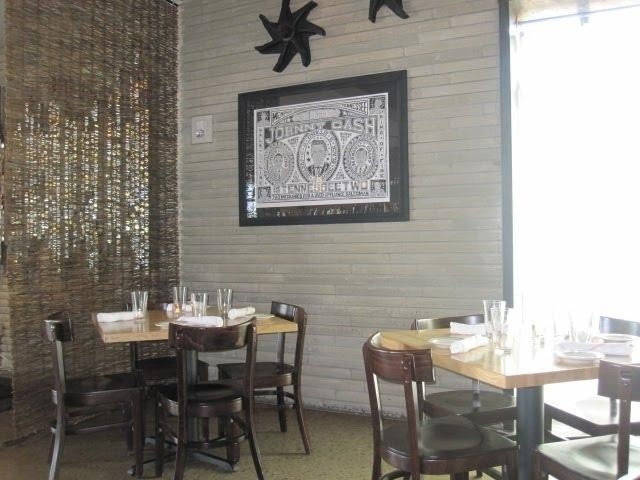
[596,333,636,343]
[153,320,171,329]
[554,349,606,364]
[425,336,465,349]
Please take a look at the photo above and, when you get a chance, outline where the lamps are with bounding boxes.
[411,315,518,479]
[156,317,264,480]
[363,331,518,480]
[533,360,640,479]
[123,300,209,451]
[217,301,310,454]
[544,316,639,443]
[43,311,147,480]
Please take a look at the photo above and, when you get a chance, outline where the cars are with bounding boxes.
[216,287,234,323]
[483,299,507,346]
[171,286,186,320]
[191,291,209,324]
[491,306,517,352]
[130,290,149,322]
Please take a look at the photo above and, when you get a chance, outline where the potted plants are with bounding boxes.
[238,71,409,227]
